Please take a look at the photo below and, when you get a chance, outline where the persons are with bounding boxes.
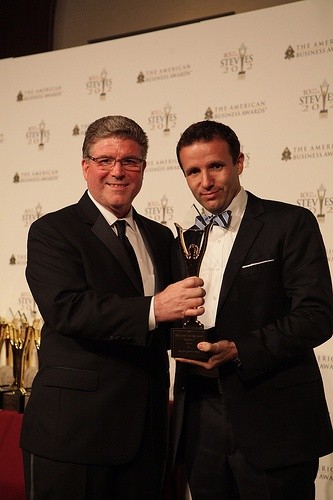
[20,116,206,498]
[173,121,333,500]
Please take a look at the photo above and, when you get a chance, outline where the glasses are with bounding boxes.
[88,155,144,171]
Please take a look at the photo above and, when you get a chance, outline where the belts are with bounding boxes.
[185,377,223,394]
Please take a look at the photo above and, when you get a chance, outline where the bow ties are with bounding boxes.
[195,210,232,230]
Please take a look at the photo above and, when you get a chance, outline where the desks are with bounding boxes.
[0,401,174,500]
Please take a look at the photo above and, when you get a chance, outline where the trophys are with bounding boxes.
[0,324,35,410]
[171,222,215,362]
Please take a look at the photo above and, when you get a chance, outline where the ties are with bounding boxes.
[114,220,130,243]
